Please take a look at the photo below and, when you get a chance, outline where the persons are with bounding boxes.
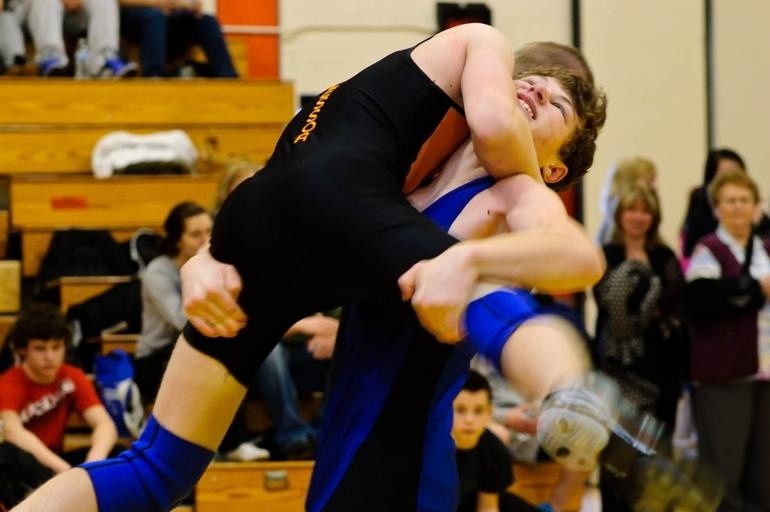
[3,1,769,512]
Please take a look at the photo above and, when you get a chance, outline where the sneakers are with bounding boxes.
[226,442,271,461]
[534,387,707,512]
[0,48,239,78]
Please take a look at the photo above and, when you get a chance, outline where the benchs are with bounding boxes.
[0,69,594,512]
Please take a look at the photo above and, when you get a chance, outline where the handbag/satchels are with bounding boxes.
[685,237,762,383]
